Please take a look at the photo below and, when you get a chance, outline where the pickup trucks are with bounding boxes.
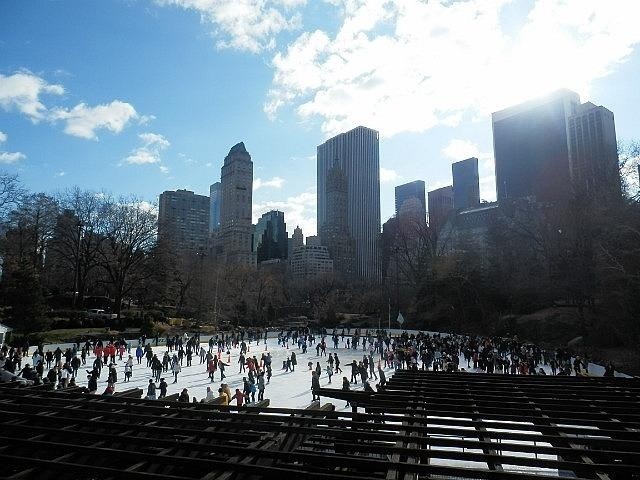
[89,309,118,319]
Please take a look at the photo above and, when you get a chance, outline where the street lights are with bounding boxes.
[195,249,207,325]
[384,274,392,329]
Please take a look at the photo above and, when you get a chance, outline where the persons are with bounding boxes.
[1,322,616,406]
